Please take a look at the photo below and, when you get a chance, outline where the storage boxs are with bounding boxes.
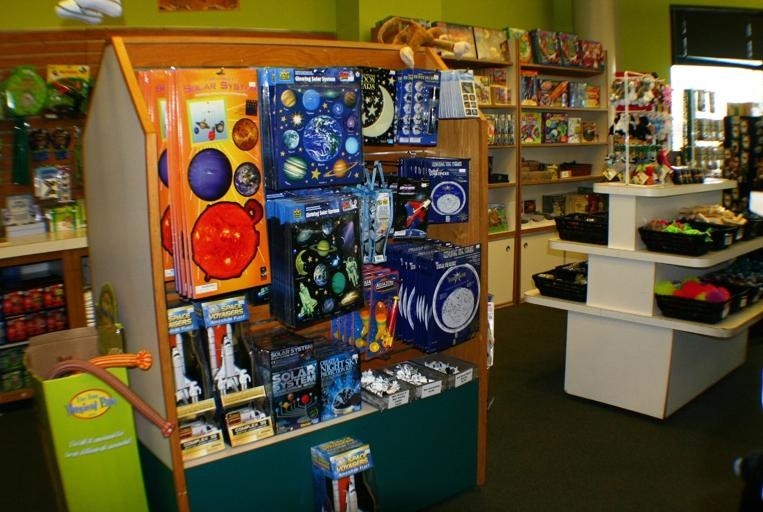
[23,326,148,512]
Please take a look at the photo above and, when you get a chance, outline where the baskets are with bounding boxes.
[679,210,763,252]
[654,280,738,323]
[701,271,763,314]
[554,212,608,246]
[531,261,588,304]
[638,219,714,257]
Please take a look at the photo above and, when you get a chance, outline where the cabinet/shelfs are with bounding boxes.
[0,247,98,404]
